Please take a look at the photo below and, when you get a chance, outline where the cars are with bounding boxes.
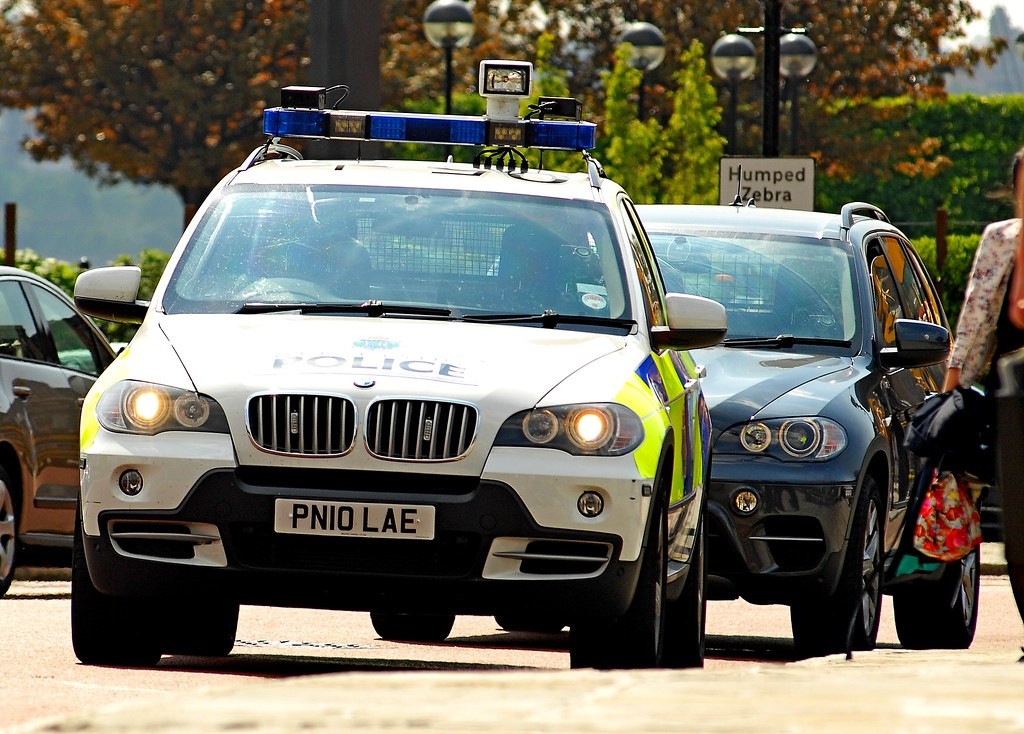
[0,264,119,593]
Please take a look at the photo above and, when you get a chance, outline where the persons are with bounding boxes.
[483,221,561,316]
[307,233,374,301]
[942,146,1024,649]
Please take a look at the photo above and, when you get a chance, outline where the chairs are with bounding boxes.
[467,226,584,314]
[291,233,390,301]
[0,311,23,359]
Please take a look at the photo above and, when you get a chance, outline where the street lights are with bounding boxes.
[620,23,665,126]
[781,34,816,158]
[425,0,477,162]
[713,35,756,161]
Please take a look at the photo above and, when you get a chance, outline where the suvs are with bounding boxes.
[486,199,988,661]
[65,62,729,671]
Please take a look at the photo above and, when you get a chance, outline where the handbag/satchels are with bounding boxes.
[899,464,983,563]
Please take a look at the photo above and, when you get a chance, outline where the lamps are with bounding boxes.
[751,258,845,341]
